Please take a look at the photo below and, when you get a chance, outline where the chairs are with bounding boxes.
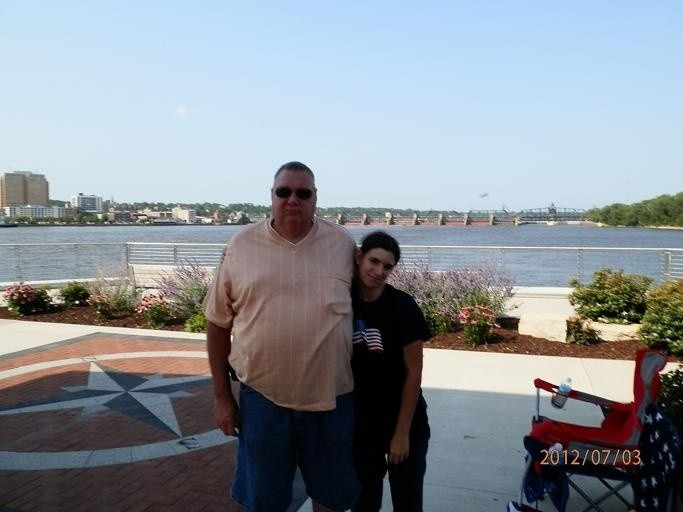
[506,349,682,511]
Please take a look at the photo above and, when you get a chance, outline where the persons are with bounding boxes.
[201,160,359,511]
[228,232,433,511]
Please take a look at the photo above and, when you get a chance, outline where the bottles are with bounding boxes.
[551,378,572,409]
[549,443,566,467]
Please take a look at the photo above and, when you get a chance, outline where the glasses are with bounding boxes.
[273,188,316,200]
